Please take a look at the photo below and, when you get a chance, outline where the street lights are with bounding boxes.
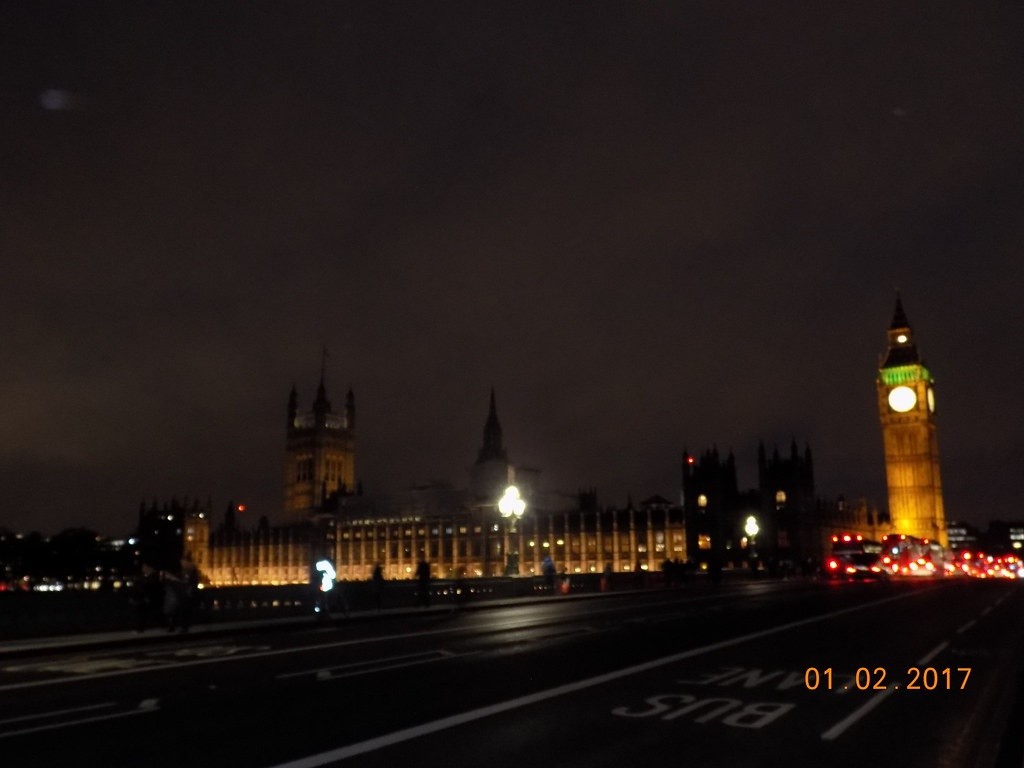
[497,486,527,575]
[745,515,759,573]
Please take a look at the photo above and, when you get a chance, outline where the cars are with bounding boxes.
[828,534,1020,585]
